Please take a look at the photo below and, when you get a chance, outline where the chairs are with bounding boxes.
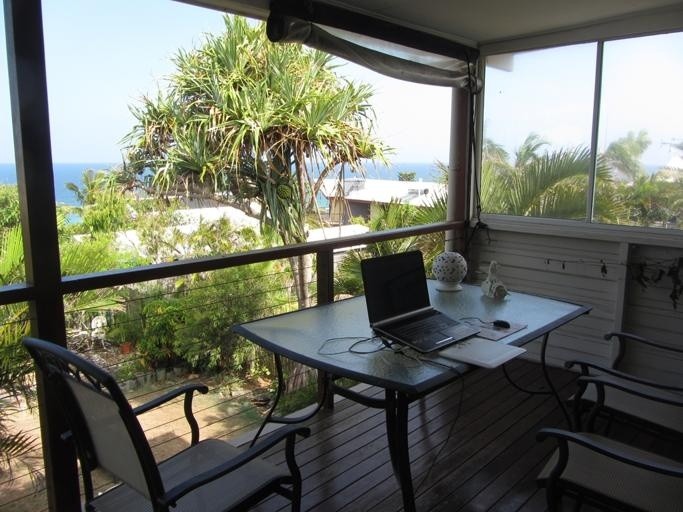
[21,333,312,511]
[561,328,680,455]
[535,375,680,512]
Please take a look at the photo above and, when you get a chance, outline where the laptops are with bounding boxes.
[360,250,481,354]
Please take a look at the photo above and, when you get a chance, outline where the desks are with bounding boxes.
[232,276,593,510]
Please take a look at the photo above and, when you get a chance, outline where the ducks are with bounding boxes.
[481,260,511,299]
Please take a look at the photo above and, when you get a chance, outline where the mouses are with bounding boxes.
[493,320,510,329]
